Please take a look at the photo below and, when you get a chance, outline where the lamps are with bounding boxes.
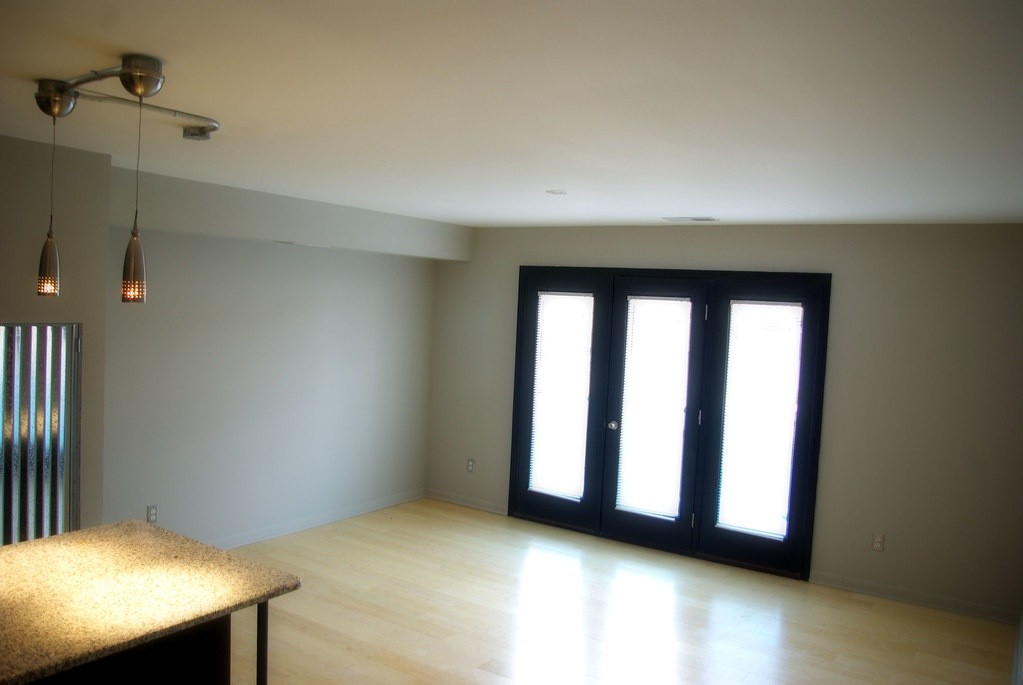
[35,53,219,306]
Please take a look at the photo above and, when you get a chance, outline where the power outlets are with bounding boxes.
[147,505,158,524]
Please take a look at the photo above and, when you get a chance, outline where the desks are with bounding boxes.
[0,522,302,685]
